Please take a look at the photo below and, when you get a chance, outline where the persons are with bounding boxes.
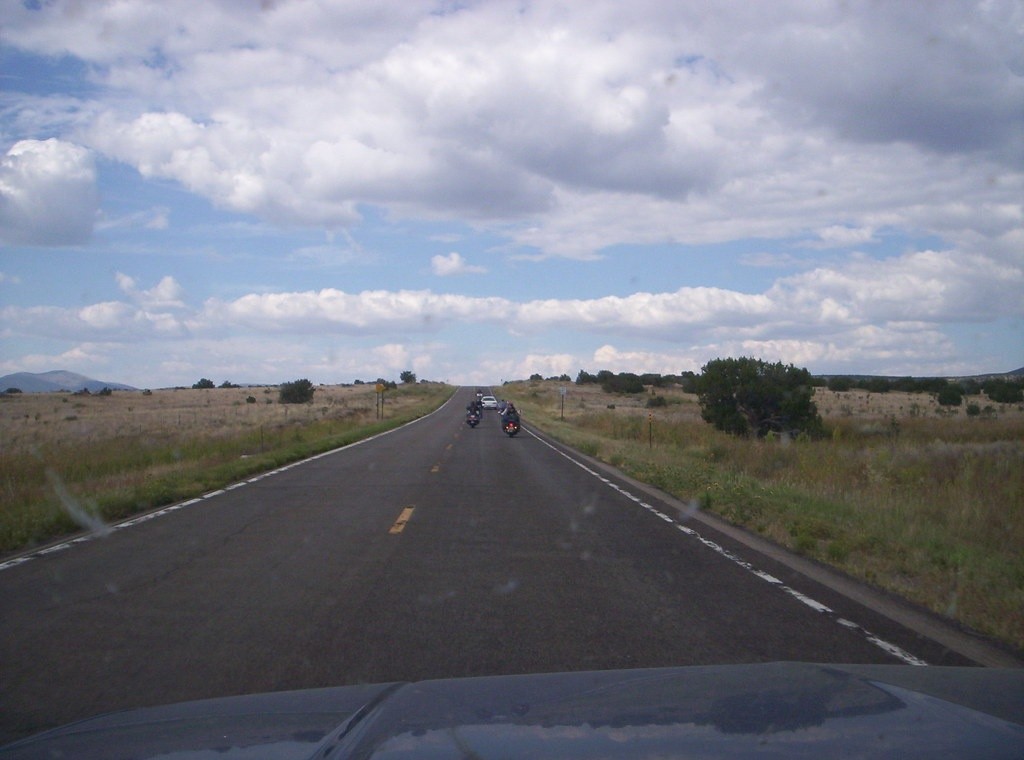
[498,399,520,429]
[467,400,484,421]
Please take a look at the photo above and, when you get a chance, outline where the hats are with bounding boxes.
[509,402,512,406]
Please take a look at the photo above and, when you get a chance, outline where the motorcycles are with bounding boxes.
[499,412,520,437]
[465,409,480,428]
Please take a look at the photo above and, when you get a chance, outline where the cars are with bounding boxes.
[481,396,497,409]
